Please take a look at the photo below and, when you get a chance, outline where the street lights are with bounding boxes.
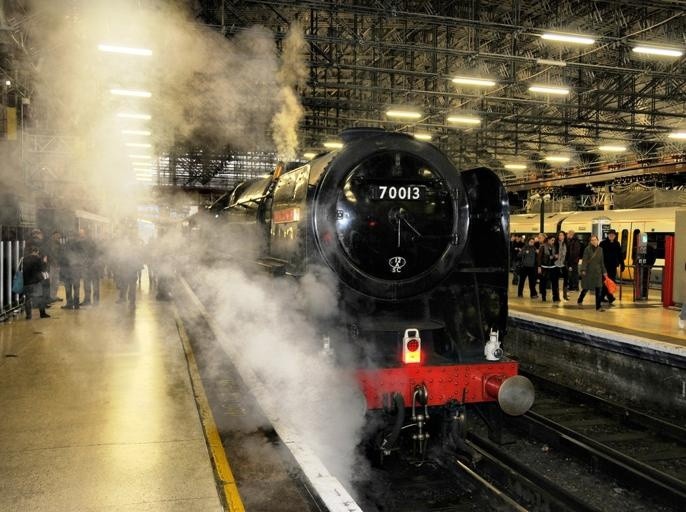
[529,194,552,234]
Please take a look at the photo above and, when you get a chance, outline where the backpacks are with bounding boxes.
[604,275,617,294]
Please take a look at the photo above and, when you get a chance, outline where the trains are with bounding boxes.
[162,127,535,469]
[509,206,686,283]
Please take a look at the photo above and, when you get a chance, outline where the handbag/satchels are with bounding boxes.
[511,274,519,287]
[10,256,26,295]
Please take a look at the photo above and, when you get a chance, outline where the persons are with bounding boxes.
[19,228,181,318]
[509,226,656,313]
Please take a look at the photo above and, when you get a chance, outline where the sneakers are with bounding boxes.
[115,297,137,310]
[518,292,570,302]
[60,298,100,310]
[25,305,52,320]
[677,317,684,328]
[577,298,617,313]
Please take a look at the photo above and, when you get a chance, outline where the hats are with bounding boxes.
[607,229,616,234]
